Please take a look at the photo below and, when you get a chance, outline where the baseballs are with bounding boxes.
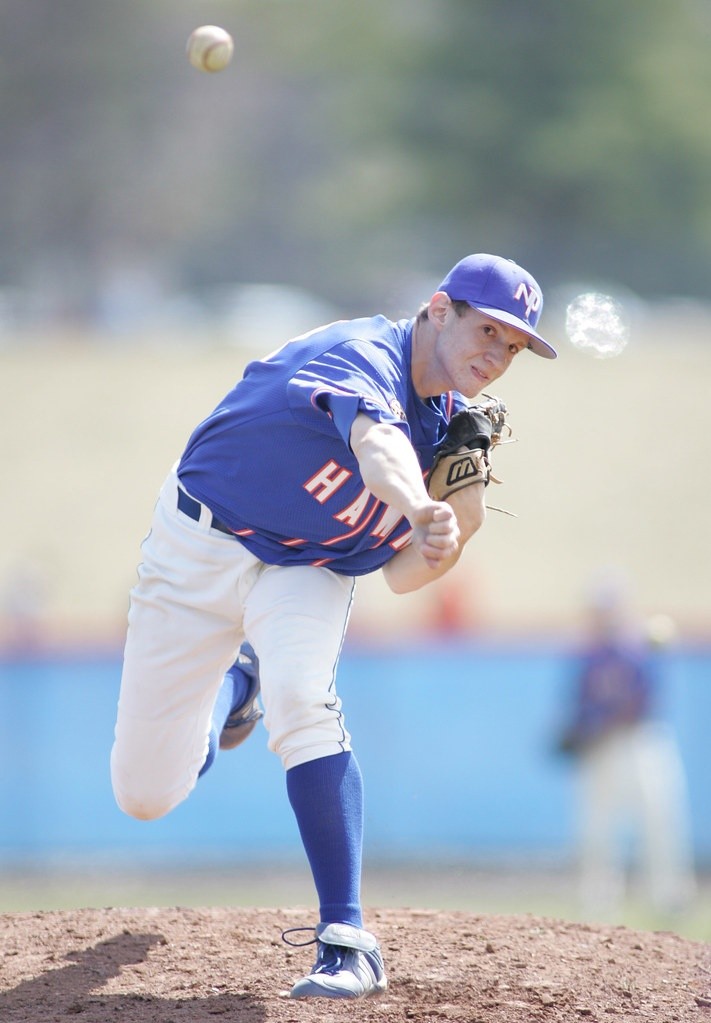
[185,23,234,74]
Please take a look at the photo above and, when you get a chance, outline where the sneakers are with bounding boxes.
[281,922,387,1002]
[219,640,264,749]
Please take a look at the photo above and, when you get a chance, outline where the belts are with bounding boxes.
[177,487,235,536]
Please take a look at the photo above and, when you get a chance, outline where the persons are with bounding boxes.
[109,251,559,999]
[560,585,695,917]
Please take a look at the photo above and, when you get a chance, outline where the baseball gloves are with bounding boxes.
[425,393,510,504]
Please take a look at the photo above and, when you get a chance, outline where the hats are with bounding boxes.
[438,252,557,359]
